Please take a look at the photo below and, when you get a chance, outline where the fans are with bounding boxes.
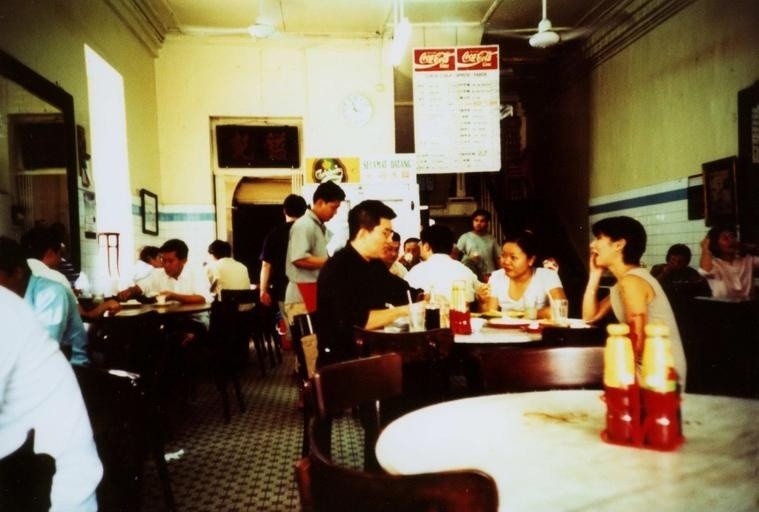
[181,0,324,46]
[485,1,602,52]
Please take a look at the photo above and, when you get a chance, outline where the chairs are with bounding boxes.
[220,288,285,378]
[296,281,320,330]
[181,301,247,426]
[485,346,604,390]
[293,416,499,511]
[301,352,401,470]
[356,328,454,400]
[70,363,175,512]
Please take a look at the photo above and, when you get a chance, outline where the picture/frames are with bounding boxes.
[698,153,739,232]
[139,187,161,236]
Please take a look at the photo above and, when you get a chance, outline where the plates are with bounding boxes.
[90,289,179,306]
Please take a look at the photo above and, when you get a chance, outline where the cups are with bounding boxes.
[408,281,588,338]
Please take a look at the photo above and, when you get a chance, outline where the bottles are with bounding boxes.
[635,322,682,449]
[603,325,640,444]
